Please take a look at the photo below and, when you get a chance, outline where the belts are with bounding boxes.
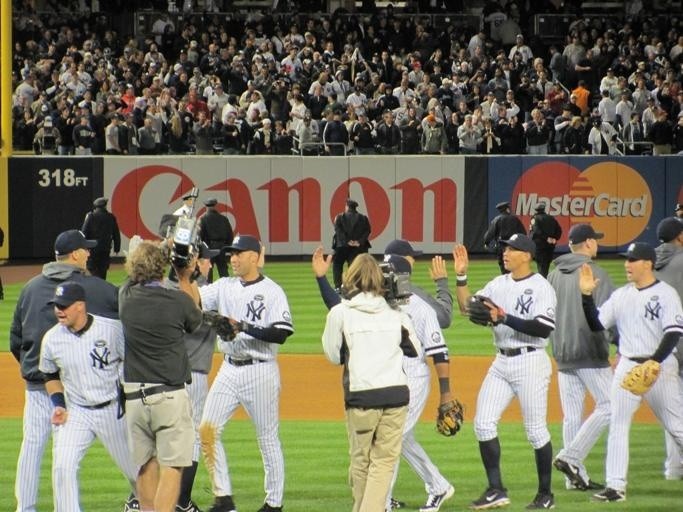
[631,357,651,366]
[224,354,267,367]
[498,346,536,357]
[81,400,111,411]
[122,381,184,399]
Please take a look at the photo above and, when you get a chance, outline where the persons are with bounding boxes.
[483,201,528,275]
[118,240,202,509]
[313,245,464,511]
[1,0,683,156]
[332,200,372,292]
[579,244,681,502]
[173,194,245,510]
[543,224,620,489]
[384,240,452,329]
[199,235,294,509]
[36,282,138,510]
[529,203,561,280]
[12,230,119,512]
[83,198,121,280]
[650,216,680,294]
[321,254,421,511]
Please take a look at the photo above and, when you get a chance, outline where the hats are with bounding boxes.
[500,215,683,262]
[204,197,216,206]
[346,199,358,208]
[199,242,220,260]
[534,202,546,212]
[384,255,411,275]
[93,197,109,207]
[183,194,197,200]
[385,240,422,257]
[46,282,86,307]
[221,234,260,254]
[495,202,510,212]
[54,230,97,256]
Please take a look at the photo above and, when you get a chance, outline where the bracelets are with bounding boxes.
[457,275,468,280]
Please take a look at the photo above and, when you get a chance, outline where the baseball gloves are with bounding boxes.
[215,315,243,343]
[467,297,507,326]
[437,398,462,436]
[620,359,661,397]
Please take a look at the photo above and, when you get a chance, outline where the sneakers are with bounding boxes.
[389,498,405,509]
[174,500,201,512]
[468,487,511,509]
[419,484,455,511]
[333,284,344,294]
[565,477,605,490]
[525,492,555,510]
[552,457,591,491]
[593,488,627,504]
[123,497,140,511]
[207,497,236,512]
